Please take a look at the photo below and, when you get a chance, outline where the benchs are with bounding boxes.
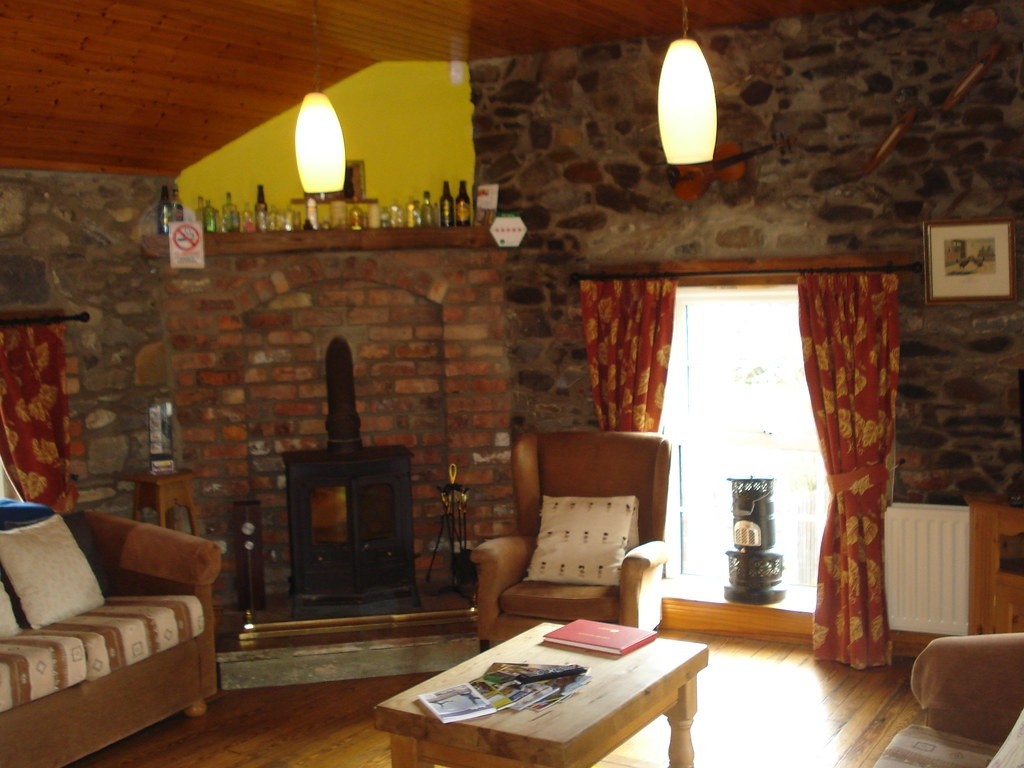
[0,509,221,768]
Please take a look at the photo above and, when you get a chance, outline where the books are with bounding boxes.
[543,617,657,656]
[417,662,592,724]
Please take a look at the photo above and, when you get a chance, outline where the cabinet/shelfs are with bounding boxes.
[968,493,1024,635]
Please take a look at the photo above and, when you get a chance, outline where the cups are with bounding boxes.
[331,201,347,230]
[367,204,379,229]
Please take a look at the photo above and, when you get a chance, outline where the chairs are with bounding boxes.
[470,427,672,652]
[869,630,1024,768]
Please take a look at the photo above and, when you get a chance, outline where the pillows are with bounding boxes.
[0,581,23,638]
[0,513,105,631]
[523,494,640,586]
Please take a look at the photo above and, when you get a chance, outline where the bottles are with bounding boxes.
[255,185,268,232]
[380,208,390,228]
[391,199,405,228]
[440,181,455,228]
[304,193,319,231]
[407,197,424,228]
[421,191,439,228]
[349,198,364,230]
[270,207,301,231]
[158,185,173,233]
[170,189,183,221]
[242,203,254,233]
[195,196,222,233]
[221,193,240,233]
[456,181,470,227]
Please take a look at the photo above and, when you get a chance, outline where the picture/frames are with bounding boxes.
[921,217,1017,307]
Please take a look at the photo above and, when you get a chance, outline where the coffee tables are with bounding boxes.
[374,621,709,768]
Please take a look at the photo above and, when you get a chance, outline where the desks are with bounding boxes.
[122,469,202,539]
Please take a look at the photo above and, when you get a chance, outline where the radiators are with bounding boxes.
[885,502,970,636]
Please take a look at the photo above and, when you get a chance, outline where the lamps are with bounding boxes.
[293,0,346,194]
[658,0,718,165]
